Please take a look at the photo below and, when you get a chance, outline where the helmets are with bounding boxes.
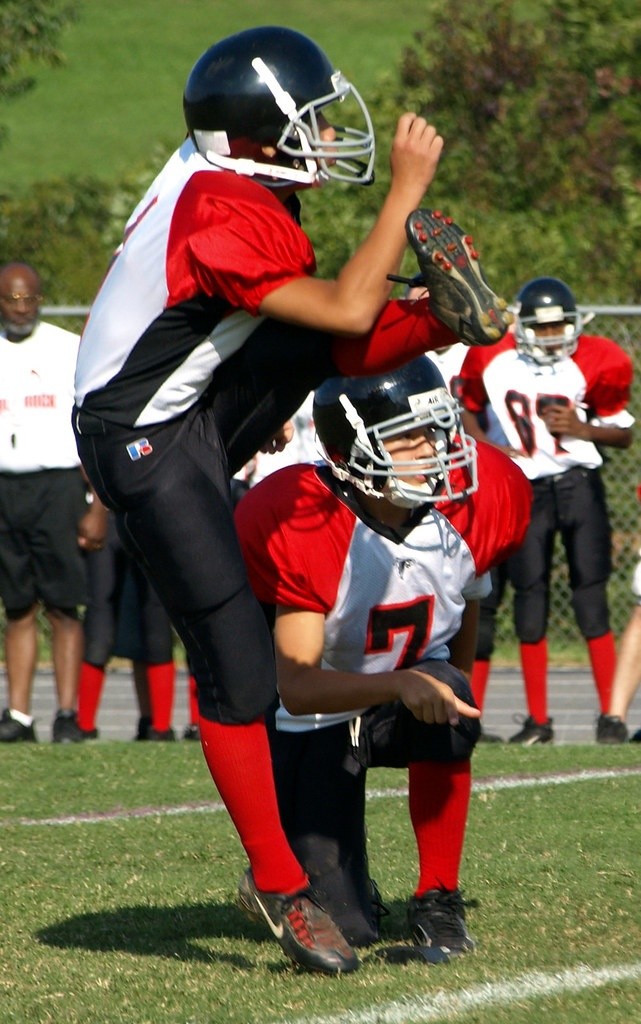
[518,277,584,366]
[183,24,375,188]
[313,354,478,508]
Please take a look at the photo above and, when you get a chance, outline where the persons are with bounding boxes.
[232,356,535,953]
[406,273,641,747]
[71,26,516,977]
[0,262,200,744]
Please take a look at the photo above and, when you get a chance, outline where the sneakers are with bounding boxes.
[405,208,514,346]
[508,715,553,746]
[407,876,479,964]
[54,709,82,744]
[598,716,627,742]
[239,868,358,973]
[0,709,35,742]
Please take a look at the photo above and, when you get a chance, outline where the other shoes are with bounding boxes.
[185,726,200,739]
[139,720,173,741]
